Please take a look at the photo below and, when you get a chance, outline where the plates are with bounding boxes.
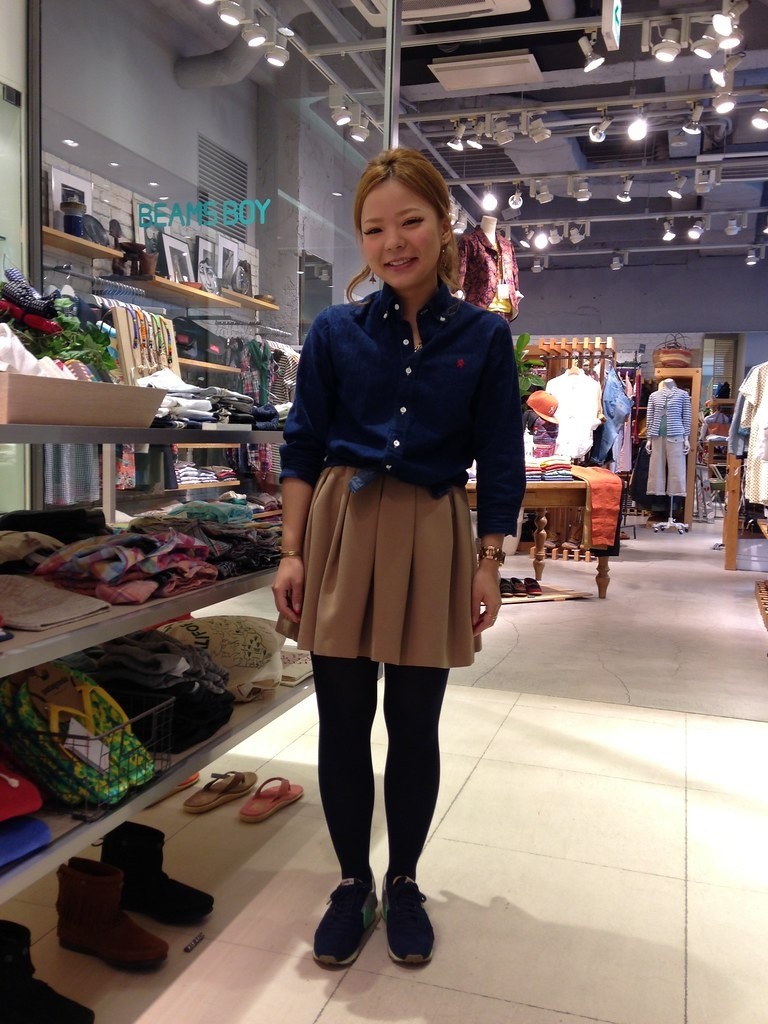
[199,260,223,296]
[82,214,110,247]
[179,280,202,289]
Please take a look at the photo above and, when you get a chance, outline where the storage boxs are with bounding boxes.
[0,371,168,427]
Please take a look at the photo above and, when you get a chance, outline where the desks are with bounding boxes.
[465,479,627,597]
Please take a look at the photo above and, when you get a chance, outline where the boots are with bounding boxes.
[55,857,168,964]
[0,920,95,1024]
[91,820,214,920]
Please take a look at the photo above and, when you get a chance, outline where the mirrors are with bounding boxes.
[300,248,333,344]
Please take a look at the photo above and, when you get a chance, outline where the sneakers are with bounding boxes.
[312,865,378,965]
[381,872,435,962]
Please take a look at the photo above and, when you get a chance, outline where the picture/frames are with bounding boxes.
[195,236,221,296]
[158,231,195,284]
[132,198,173,276]
[216,233,238,290]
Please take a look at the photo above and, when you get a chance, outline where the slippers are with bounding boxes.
[145,770,200,809]
[183,771,258,813]
[239,777,303,823]
[0,660,155,804]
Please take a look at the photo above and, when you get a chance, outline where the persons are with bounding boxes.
[646,377,692,496]
[452,216,526,323]
[198,260,217,293]
[278,149,527,965]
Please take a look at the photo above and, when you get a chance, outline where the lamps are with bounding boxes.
[446,0,768,274]
[217,0,371,142]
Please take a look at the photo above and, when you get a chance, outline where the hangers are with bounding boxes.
[51,262,293,346]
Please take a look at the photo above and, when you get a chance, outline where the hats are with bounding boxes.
[526,390,559,424]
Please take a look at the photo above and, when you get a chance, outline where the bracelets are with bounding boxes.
[280,549,303,559]
[478,546,506,567]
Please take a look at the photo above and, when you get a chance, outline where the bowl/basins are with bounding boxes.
[254,293,276,305]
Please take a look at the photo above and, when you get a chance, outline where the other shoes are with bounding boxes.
[499,578,513,596]
[510,577,527,595]
[524,578,542,594]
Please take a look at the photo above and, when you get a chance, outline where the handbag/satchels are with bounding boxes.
[156,615,287,701]
[653,332,699,368]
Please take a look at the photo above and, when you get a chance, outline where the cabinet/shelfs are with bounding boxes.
[464,335,768,630]
[0,424,321,906]
